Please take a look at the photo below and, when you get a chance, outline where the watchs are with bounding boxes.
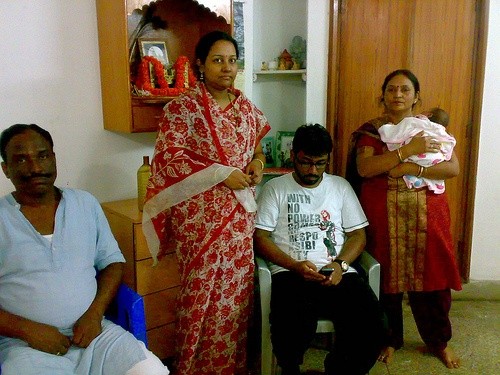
[254,158,265,170]
[334,258,348,272]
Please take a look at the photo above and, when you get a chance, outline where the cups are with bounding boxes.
[268,62,278,70]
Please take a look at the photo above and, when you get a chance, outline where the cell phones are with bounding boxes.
[319,268,335,276]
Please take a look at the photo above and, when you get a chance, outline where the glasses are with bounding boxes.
[295,154,330,167]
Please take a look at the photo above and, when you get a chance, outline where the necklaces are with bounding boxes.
[218,91,240,132]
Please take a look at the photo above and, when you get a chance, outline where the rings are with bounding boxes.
[56,352,60,356]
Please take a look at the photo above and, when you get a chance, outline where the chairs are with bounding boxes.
[254,249,380,375]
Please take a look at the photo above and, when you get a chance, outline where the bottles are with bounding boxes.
[137,156,152,211]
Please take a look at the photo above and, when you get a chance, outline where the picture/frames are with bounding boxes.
[137,36,170,67]
[259,130,296,168]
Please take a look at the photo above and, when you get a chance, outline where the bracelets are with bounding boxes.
[396,147,404,163]
[416,166,426,178]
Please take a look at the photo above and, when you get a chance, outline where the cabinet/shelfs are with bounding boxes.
[101,199,183,358]
[96,0,235,134]
[252,0,308,176]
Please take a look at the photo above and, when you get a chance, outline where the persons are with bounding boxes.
[352,63,464,370]
[146,31,271,375]
[394,108,450,193]
[255,123,392,375]
[260,143,271,163]
[0,124,172,374]
